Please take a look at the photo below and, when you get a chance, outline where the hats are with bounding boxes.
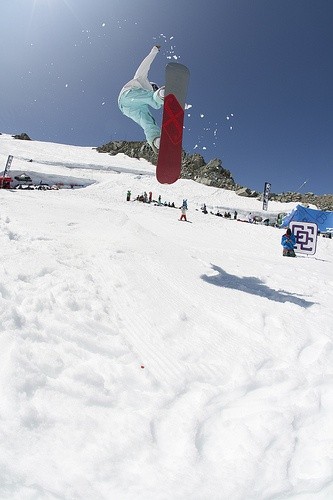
[287,229,291,235]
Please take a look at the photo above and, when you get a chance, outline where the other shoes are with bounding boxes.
[159,89,165,101]
[153,138,160,148]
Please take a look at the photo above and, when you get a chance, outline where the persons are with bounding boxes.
[14,179,59,190]
[117,45,166,155]
[179,206,187,221]
[281,228,296,257]
[126,190,252,224]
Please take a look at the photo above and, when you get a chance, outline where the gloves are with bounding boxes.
[156,45,161,51]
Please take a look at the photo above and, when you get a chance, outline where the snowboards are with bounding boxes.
[156,62,190,185]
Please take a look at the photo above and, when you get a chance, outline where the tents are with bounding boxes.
[0,173,32,188]
